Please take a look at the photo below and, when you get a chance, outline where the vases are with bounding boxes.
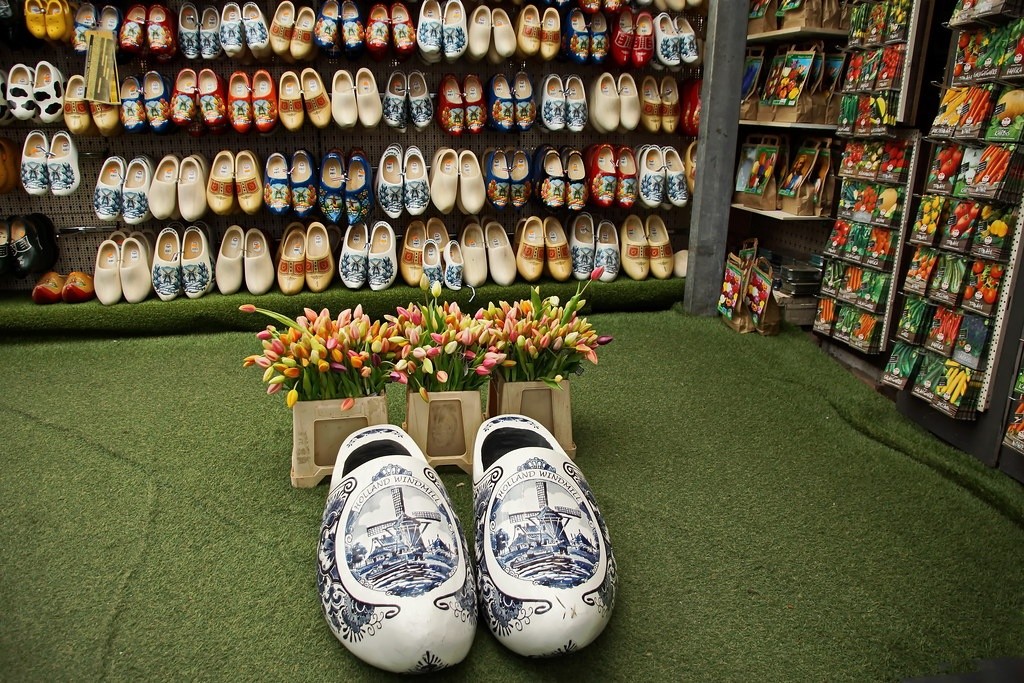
[481,368,578,460]
[401,389,481,475]
[290,386,392,488]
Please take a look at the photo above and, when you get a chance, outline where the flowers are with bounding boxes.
[479,266,612,389]
[382,271,516,403]
[239,303,408,411]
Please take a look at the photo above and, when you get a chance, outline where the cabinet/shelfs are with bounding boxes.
[729,24,849,326]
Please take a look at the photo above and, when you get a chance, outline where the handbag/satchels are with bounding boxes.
[740,38,850,125]
[748,0,859,36]
[717,240,781,336]
[735,134,840,217]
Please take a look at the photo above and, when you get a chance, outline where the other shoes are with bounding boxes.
[317,424,476,671]
[0,0,706,305]
[470,415,616,659]
